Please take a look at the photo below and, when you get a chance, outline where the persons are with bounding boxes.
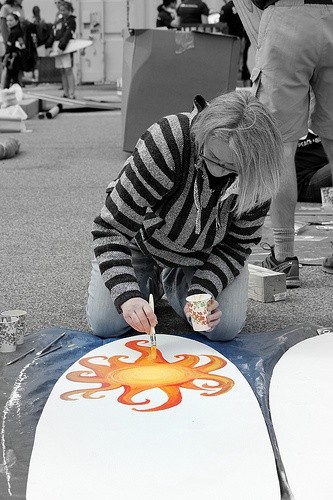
[85,91,284,342]
[0,0,253,100]
[249,0,333,289]
[294,85,333,204]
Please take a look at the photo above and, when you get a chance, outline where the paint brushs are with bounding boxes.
[35,332,66,358]
[149,294,157,359]
[7,348,35,364]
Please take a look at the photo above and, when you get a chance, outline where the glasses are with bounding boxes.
[199,146,238,174]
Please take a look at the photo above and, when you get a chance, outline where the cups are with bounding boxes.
[321,187,333,207]
[186,294,211,331]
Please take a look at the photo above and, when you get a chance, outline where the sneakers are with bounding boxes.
[252,241,301,287]
[321,240,333,274]
